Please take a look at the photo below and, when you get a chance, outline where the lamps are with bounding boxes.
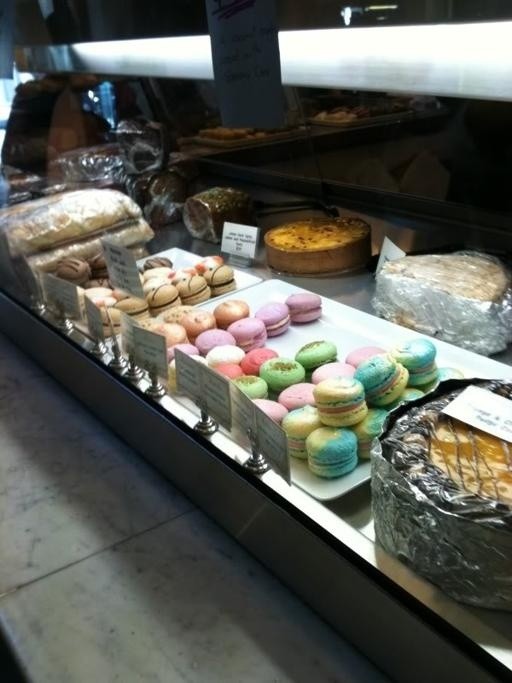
[340,3,398,25]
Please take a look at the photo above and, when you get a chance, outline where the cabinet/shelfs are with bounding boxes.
[2,1,510,682]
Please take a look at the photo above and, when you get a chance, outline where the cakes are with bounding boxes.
[146,285,182,317]
[278,382,317,410]
[180,309,217,343]
[255,302,291,337]
[203,264,236,295]
[422,367,464,396]
[112,296,151,320]
[240,348,279,376]
[56,255,89,285]
[312,362,355,384]
[168,355,208,395]
[195,327,236,355]
[391,338,439,386]
[251,399,288,425]
[387,387,425,412]
[86,249,108,278]
[282,405,322,459]
[394,381,512,524]
[263,214,371,275]
[176,275,211,305]
[345,346,385,368]
[152,323,190,349]
[206,344,245,369]
[353,353,409,408]
[295,341,337,378]
[305,426,358,479]
[259,357,306,401]
[284,293,322,323]
[211,364,245,379]
[312,376,368,426]
[165,343,199,362]
[353,407,388,460]
[227,316,267,353]
[99,307,121,336]
[213,299,249,331]
[233,375,270,401]
